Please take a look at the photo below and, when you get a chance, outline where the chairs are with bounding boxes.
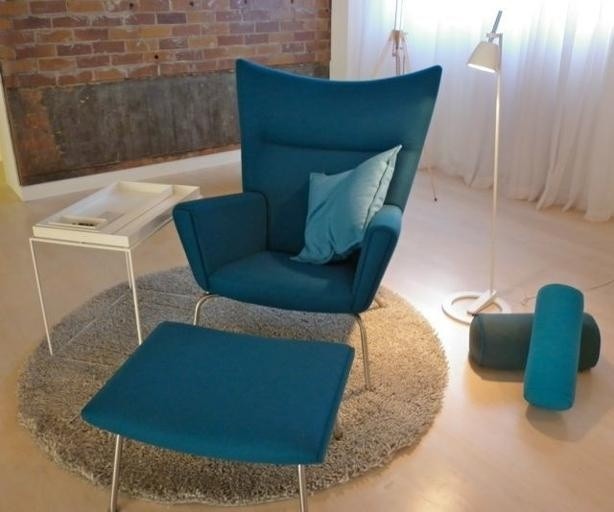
[171,57,443,392]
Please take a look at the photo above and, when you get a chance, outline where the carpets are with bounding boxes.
[13,265,448,503]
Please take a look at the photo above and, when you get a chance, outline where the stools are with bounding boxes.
[78,323,358,511]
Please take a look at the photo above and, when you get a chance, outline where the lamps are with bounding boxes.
[440,11,510,323]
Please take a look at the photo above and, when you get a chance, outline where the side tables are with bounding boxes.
[23,179,203,355]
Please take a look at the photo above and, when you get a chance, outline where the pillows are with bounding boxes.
[469,283,599,412]
[289,142,402,265]
